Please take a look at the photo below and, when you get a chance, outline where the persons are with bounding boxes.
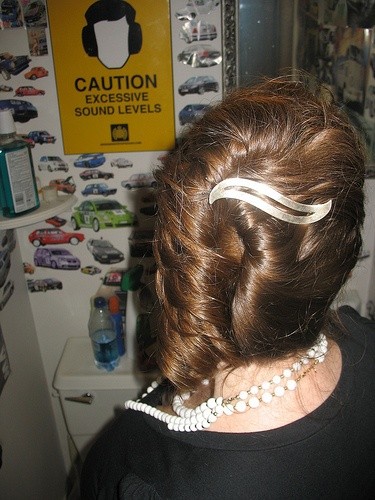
[65,70,375,500]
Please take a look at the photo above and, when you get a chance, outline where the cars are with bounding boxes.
[23,0,45,23]
[26,66,48,81]
[28,277,63,294]
[178,103,216,125]
[77,266,99,277]
[0,0,23,24]
[77,169,112,179]
[0,52,31,81]
[0,99,39,123]
[86,235,126,264]
[33,246,82,271]
[12,134,38,146]
[175,0,220,21]
[21,259,36,274]
[178,46,222,67]
[110,159,132,167]
[27,225,87,250]
[81,182,118,196]
[180,18,217,43]
[74,152,106,167]
[176,75,220,96]
[45,215,66,229]
[16,84,45,96]
[69,198,137,233]
[45,173,77,196]
[37,154,68,173]
[31,129,55,142]
[121,172,158,190]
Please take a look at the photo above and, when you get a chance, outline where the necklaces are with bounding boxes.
[123,333,328,432]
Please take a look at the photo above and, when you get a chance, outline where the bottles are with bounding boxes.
[89,298,120,371]
[109,297,124,354]
[1,110,41,217]
